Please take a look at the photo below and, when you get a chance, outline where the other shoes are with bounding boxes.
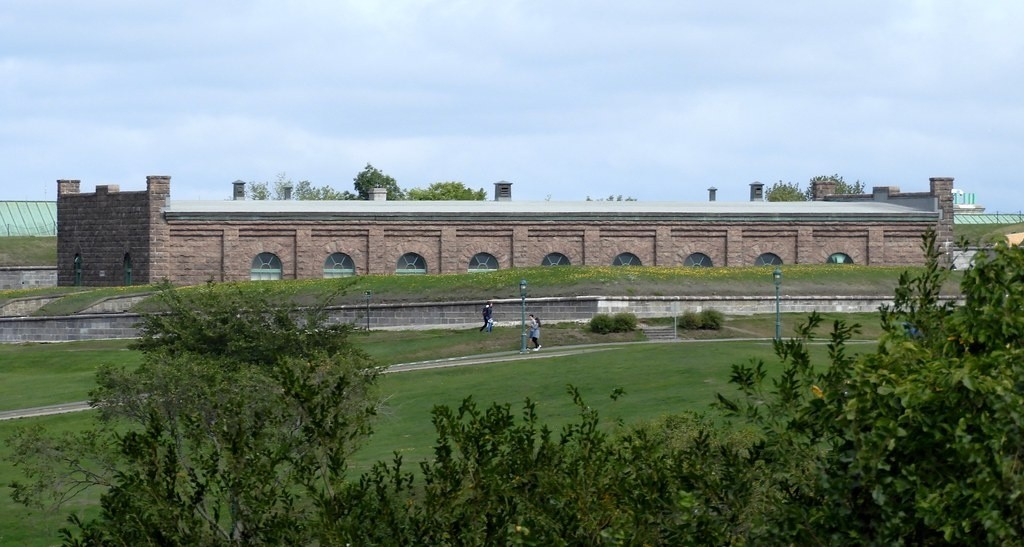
[533,347,540,351]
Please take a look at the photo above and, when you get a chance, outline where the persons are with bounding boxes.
[526,314,537,351]
[480,301,493,332]
[531,317,541,351]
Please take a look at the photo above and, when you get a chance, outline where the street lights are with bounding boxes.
[773,265,782,342]
[520,279,528,352]
